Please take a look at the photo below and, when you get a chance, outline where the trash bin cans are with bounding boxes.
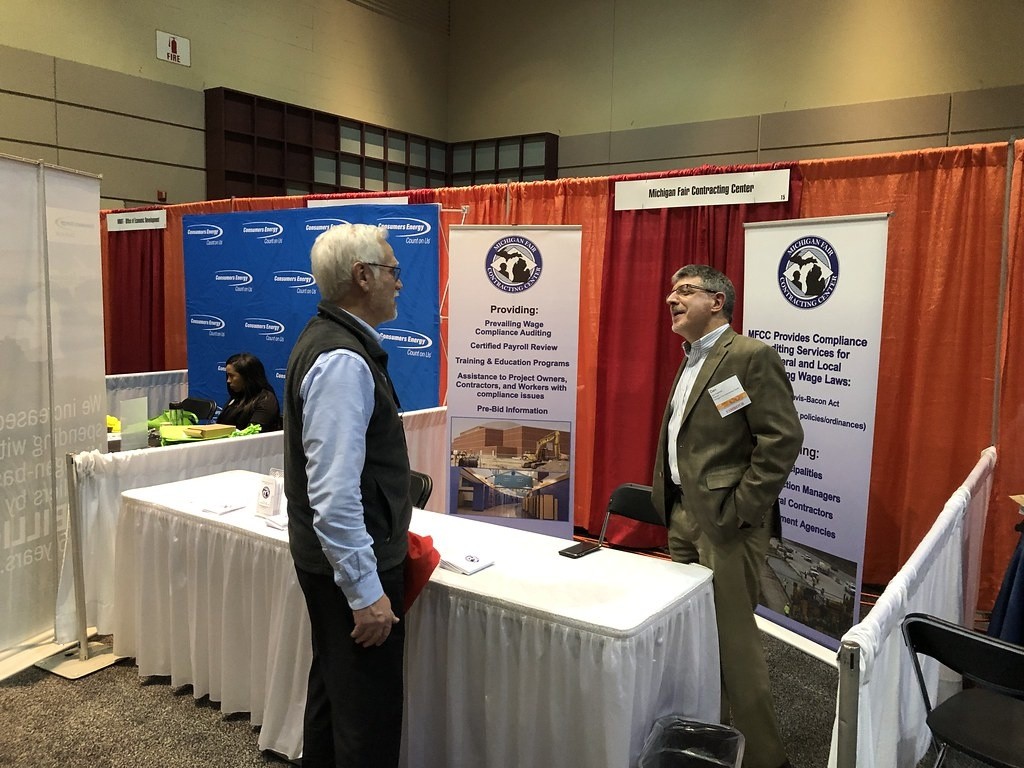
[636,713,746,768]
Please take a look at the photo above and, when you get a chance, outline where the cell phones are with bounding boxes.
[559,541,601,559]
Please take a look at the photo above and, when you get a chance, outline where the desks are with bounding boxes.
[119,470,716,768]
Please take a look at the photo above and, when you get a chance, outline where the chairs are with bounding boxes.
[598,481,670,561]
[898,612,1024,768]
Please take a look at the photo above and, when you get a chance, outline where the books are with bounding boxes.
[204,501,247,515]
[266,515,290,531]
[440,545,496,575]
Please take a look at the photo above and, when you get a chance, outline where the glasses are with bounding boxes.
[362,263,401,282]
[666,284,716,301]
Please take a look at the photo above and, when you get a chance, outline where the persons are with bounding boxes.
[286,222,412,768]
[653,262,805,767]
[217,351,282,432]
